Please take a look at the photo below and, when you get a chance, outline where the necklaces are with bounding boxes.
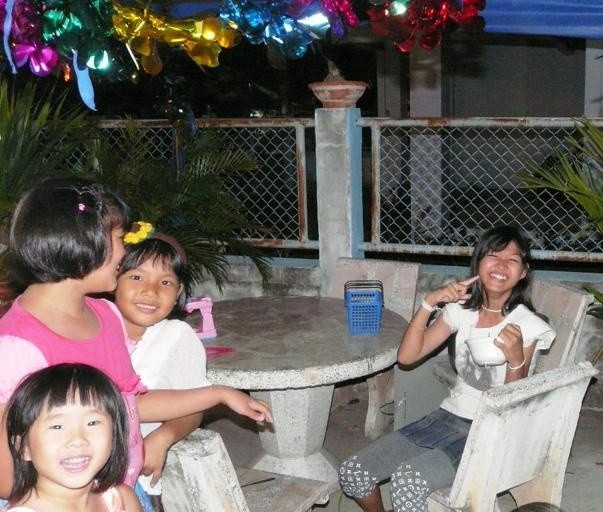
[481,303,509,313]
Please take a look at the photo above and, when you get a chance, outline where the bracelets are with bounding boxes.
[420,295,438,312]
[507,354,527,370]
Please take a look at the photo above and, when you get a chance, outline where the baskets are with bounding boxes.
[344,280,384,335]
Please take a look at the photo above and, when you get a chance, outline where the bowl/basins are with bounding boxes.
[465,337,506,366]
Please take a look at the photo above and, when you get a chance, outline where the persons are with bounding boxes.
[0,176,275,512]
[107,220,215,512]
[5,360,144,512]
[335,224,558,512]
